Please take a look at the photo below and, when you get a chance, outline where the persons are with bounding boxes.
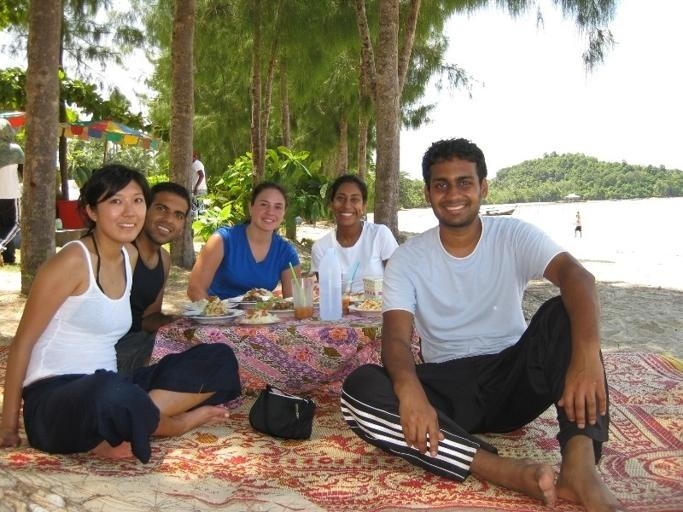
[186,182,301,303]
[1,163,241,465]
[193,150,207,196]
[311,175,399,293]
[116,180,192,391]
[340,139,629,512]
[0,119,25,266]
[572,206,585,238]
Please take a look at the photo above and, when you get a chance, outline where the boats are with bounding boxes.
[481,205,517,216]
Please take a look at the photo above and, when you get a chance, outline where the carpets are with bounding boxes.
[0,344,683,511]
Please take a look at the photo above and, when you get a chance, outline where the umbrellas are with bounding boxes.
[55,118,166,170]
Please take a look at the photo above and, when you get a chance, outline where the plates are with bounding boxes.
[346,294,381,316]
[179,295,294,324]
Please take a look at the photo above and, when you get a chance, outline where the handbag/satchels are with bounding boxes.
[247,384,316,441]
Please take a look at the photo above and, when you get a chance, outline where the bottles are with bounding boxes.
[317,248,342,321]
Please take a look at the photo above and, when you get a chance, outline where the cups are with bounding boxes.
[341,279,352,316]
[291,278,314,320]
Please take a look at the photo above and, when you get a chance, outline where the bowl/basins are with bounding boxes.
[57,200,91,229]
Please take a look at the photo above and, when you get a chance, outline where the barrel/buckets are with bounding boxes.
[56,198,87,229]
